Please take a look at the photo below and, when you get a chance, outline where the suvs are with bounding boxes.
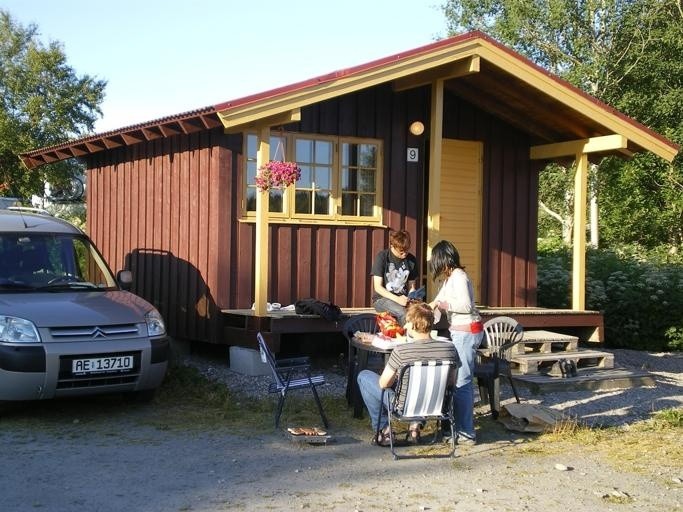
[0,206,172,401]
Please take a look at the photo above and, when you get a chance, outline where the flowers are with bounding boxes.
[253,160,302,194]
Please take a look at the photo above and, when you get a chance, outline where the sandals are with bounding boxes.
[400,428,420,444]
[369,430,398,446]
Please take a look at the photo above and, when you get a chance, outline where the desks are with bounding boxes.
[351,329,490,418]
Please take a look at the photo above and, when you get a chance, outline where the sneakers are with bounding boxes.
[441,430,476,446]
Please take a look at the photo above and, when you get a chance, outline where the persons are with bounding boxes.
[426,239,485,446]
[356,300,463,447]
[368,229,418,330]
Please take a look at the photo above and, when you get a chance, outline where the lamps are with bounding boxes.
[409,121,425,136]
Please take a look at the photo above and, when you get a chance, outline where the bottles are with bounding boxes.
[409,284,416,293]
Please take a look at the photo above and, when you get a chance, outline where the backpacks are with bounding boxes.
[293,296,348,325]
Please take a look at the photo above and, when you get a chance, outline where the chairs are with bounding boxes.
[475,316,523,418]
[0,250,53,271]
[374,360,462,461]
[257,331,330,429]
[342,314,398,404]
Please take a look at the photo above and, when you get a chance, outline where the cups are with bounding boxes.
[272,303,281,310]
[430,330,438,338]
